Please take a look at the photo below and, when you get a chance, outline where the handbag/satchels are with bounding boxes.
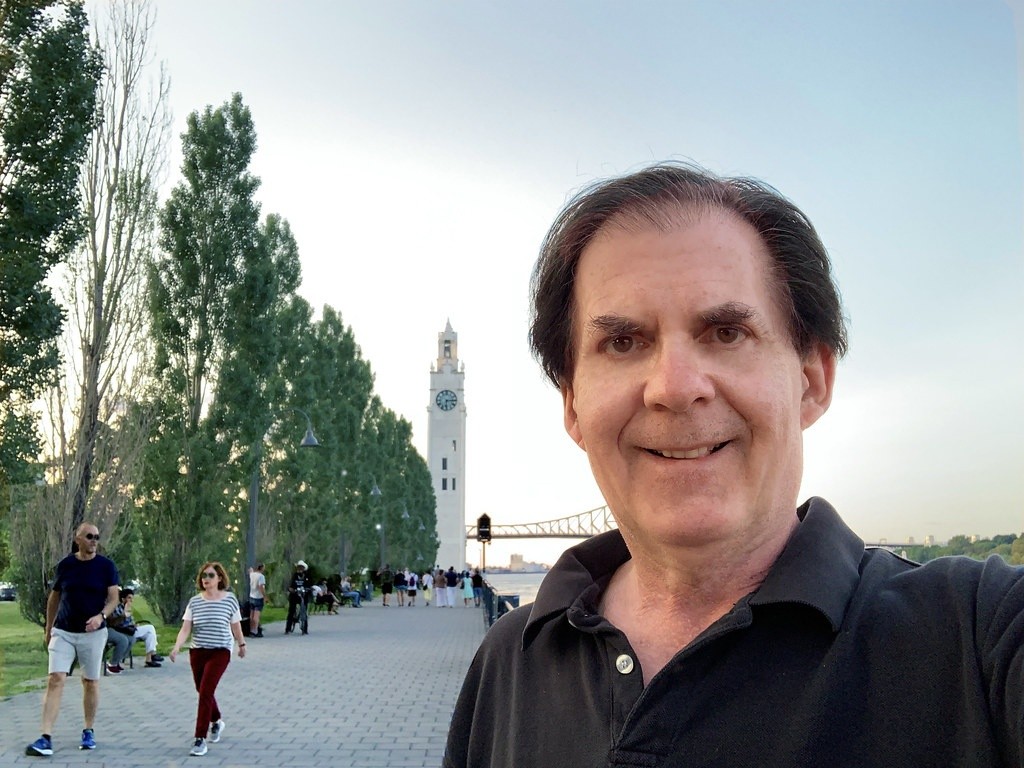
[116,624,136,636]
[423,584,427,591]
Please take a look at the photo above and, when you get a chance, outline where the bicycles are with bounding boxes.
[289,587,311,635]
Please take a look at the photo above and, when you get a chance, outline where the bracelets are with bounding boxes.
[239,643,246,647]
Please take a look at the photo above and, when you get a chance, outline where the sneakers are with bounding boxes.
[25,736,53,756]
[79,729,96,749]
[189,737,208,756]
[208,719,225,743]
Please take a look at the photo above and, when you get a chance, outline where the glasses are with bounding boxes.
[86,533,100,540]
[201,573,215,578]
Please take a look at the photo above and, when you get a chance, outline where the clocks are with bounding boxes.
[436,389,458,412]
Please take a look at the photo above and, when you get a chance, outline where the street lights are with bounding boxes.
[380,498,410,579]
[336,471,383,576]
[244,406,320,604]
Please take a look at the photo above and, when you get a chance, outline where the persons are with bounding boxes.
[283,560,311,635]
[170,562,247,756]
[404,570,419,606]
[394,568,408,606]
[434,569,446,608]
[248,563,269,638]
[312,578,340,615]
[460,572,474,608]
[430,564,474,582]
[379,563,394,607]
[421,570,433,606]
[340,576,364,608]
[438,159,1024,768]
[112,589,165,668]
[444,566,459,608]
[26,520,123,756]
[471,570,483,606]
[104,586,137,675]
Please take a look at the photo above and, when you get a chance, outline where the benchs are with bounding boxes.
[67,618,153,677]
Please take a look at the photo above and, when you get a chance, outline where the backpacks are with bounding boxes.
[410,577,415,586]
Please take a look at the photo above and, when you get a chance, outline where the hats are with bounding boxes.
[293,560,308,571]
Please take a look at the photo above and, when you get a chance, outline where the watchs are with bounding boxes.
[99,611,107,620]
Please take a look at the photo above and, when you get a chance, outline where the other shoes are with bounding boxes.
[151,655,165,661]
[145,660,161,668]
[107,661,124,674]
[249,631,263,637]
